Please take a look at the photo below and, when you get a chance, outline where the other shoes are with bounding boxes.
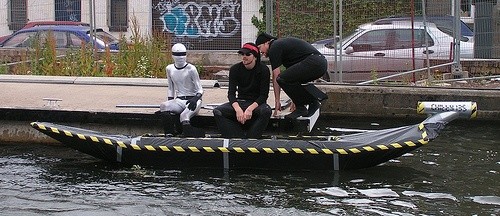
[284,106,307,119]
[180,120,198,138]
[303,100,321,116]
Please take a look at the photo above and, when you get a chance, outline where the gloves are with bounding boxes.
[186,93,202,110]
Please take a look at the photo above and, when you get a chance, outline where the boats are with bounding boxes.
[29,102,478,173]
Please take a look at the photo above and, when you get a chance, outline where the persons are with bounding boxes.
[255,32,328,120]
[213,43,272,139]
[160,43,203,137]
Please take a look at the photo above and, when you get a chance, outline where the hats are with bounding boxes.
[256,33,273,45]
[237,43,259,54]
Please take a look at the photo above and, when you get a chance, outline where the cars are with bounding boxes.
[313,15,473,43]
[309,20,473,61]
[0,25,127,59]
[0,21,133,48]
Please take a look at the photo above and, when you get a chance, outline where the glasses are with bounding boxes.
[242,53,252,56]
